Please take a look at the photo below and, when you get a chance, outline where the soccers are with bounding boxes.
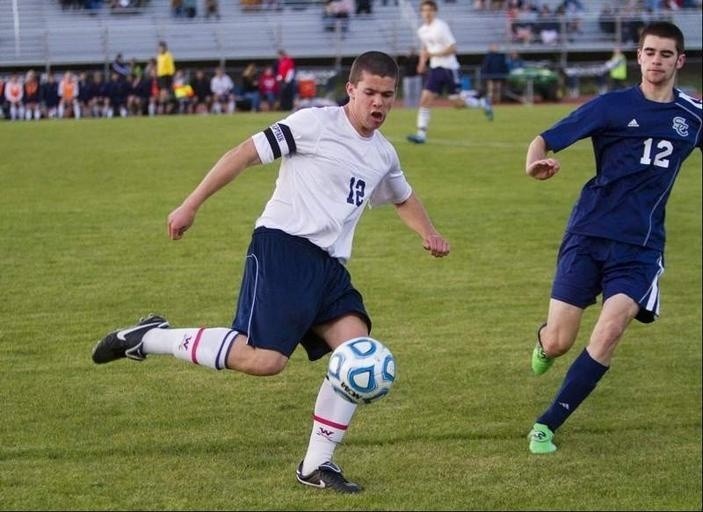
[327,337,398,407]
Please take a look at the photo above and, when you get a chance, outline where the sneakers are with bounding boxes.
[91,315,170,365]
[405,135,426,144]
[295,459,360,494]
[527,423,557,454]
[532,323,556,376]
[482,97,495,122]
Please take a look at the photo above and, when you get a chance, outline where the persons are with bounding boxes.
[0,40,348,121]
[401,49,423,108]
[406,1,494,142]
[92,51,450,493]
[524,23,703,454]
[58,1,400,40]
[439,1,685,105]
